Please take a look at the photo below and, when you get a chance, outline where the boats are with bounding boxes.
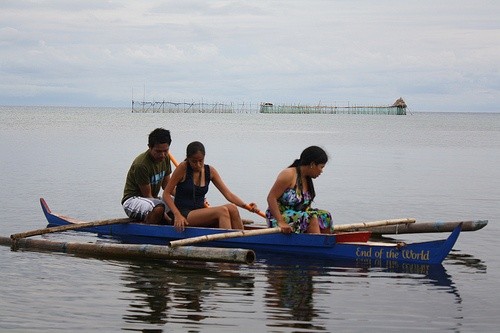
[40,194,463,275]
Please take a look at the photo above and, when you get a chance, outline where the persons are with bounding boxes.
[266,146,333,233]
[121,128,176,224]
[163,142,259,232]
